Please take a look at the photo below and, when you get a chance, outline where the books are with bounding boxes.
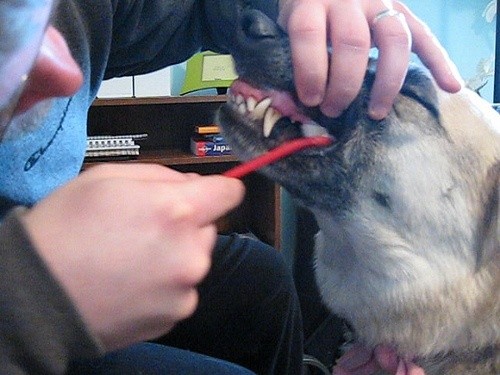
[83,132,150,163]
[202,135,225,142]
[190,138,236,157]
[194,124,222,134]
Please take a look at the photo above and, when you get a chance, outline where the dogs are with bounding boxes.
[215,9,500,375]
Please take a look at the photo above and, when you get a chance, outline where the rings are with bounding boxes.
[371,7,401,26]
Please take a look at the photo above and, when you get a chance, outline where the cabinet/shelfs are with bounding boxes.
[77,95,282,256]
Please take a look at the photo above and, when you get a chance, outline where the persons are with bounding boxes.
[0,0,463,375]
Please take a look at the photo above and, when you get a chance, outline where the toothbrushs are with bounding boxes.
[218,124,337,178]
